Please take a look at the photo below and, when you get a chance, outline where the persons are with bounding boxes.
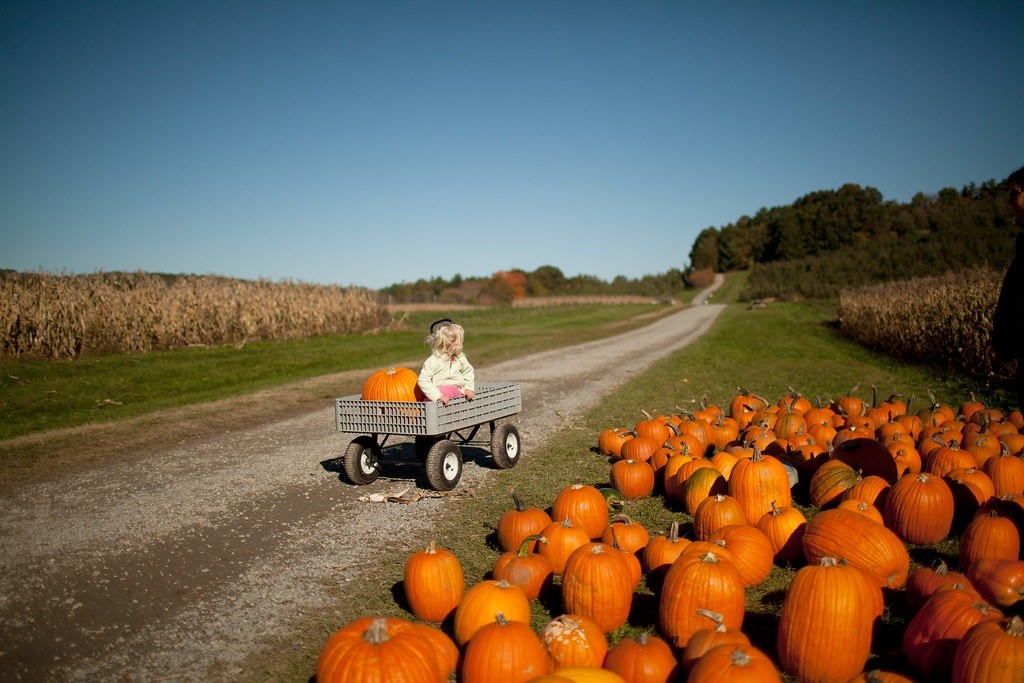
[417,318,475,405]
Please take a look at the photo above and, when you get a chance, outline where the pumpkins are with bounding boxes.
[362,367,426,402]
[316,389,1024,683]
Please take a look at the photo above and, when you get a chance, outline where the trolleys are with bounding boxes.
[336,381,523,490]
